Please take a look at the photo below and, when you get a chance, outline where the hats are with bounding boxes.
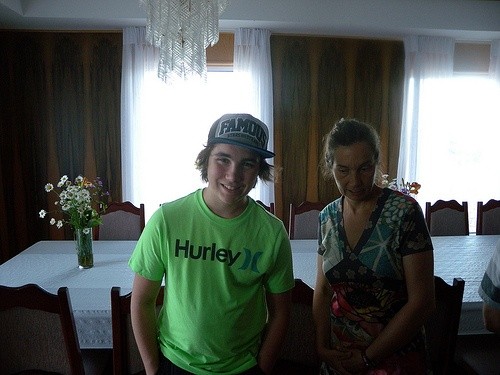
[207,113,276,159]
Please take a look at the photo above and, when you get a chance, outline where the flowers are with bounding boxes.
[39,174,111,234]
[381,174,421,196]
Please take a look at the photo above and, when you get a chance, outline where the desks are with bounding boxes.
[0,234,500,349]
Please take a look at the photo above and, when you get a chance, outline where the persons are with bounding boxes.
[314,118,434,375]
[127,114,295,375]
[477,241,500,335]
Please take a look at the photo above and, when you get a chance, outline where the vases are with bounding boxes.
[76,228,94,269]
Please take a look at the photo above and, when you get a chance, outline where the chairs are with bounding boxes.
[289,201,326,240]
[424,277,465,375]
[476,199,500,235]
[426,200,469,236]
[111,286,170,375]
[0,284,112,375]
[260,279,330,374]
[93,201,145,240]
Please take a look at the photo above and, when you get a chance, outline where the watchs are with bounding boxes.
[361,349,375,369]
